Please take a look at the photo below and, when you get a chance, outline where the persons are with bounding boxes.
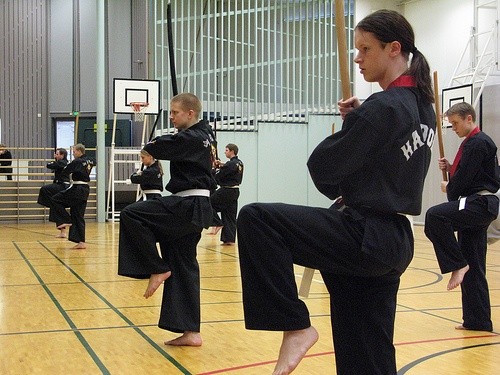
[129,148,164,202]
[236,8,437,375]
[422,103,500,331]
[207,140,244,247]
[0,143,14,181]
[48,141,97,250]
[116,92,215,347]
[36,148,70,239]
